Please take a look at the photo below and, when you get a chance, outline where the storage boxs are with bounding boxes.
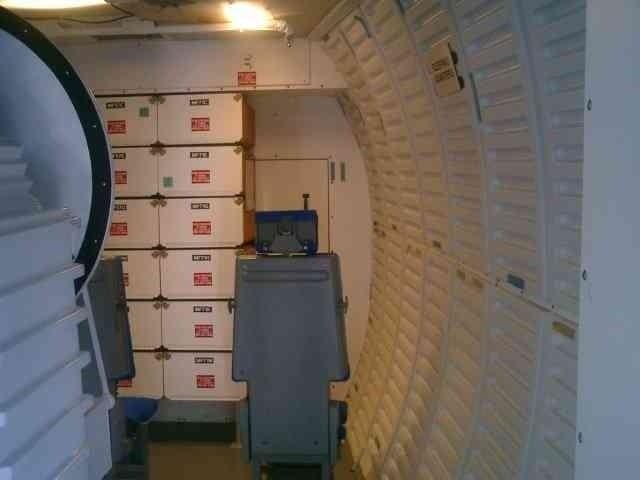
[103,200,160,250]
[157,146,244,198]
[159,196,245,250]
[161,300,235,350]
[160,248,239,302]
[164,351,248,401]
[125,302,162,352]
[156,94,251,145]
[116,354,162,400]
[93,93,157,146]
[100,251,160,300]
[111,147,157,197]
